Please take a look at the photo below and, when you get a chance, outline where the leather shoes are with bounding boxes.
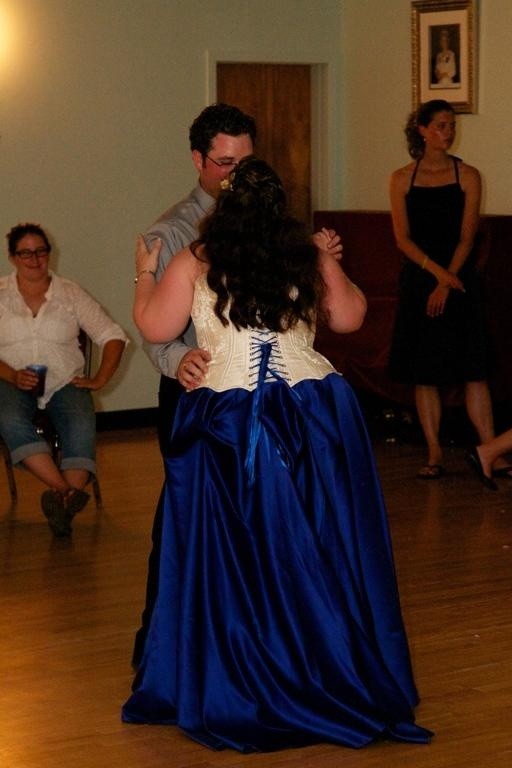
[462,446,498,490]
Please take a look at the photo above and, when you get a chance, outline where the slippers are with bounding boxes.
[420,464,441,480]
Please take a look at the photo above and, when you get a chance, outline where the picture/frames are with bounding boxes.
[411,0,480,118]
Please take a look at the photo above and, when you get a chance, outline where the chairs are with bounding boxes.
[0,330,103,508]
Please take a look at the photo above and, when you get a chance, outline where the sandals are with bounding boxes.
[40,487,91,538]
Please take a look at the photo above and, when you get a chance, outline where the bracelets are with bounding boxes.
[134,269,157,284]
[422,255,428,268]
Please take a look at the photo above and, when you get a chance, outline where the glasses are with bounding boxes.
[206,156,240,172]
[10,246,52,259]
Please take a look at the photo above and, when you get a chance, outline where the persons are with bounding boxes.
[120,155,434,753]
[473,428,512,492]
[132,102,344,670]
[434,30,456,84]
[0,223,130,539]
[390,99,512,479]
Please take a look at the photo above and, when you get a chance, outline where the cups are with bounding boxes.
[27,364,47,398]
